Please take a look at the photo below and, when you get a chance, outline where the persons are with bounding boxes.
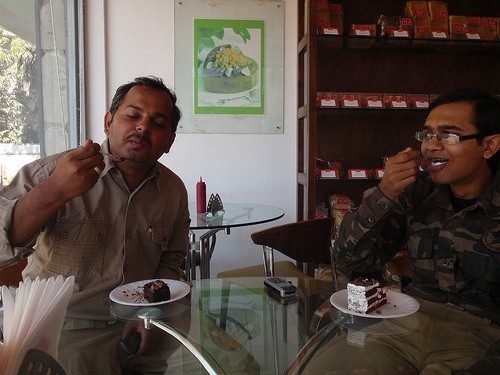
[1,78,260,374]
[285,86,500,375]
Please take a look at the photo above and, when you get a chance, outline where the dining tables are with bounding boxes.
[0,276,499,375]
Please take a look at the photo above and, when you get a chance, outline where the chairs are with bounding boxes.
[288,252,413,375]
[217,216,336,346]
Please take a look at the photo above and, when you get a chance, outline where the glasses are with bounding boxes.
[414,130,485,145]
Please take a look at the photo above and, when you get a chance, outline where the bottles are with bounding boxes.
[196,176,206,213]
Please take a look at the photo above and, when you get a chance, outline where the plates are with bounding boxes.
[108,276,191,307]
[329,287,420,318]
[109,301,189,321]
[330,309,420,334]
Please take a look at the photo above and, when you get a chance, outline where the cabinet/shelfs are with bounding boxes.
[299,1,500,276]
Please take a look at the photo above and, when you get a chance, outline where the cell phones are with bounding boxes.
[121,329,143,355]
[263,277,297,298]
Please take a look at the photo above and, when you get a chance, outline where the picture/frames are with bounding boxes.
[174,0,286,135]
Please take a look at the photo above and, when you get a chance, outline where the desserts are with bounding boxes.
[346,277,388,314]
[144,280,170,303]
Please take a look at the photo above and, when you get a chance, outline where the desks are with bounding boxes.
[187,202,285,352]
